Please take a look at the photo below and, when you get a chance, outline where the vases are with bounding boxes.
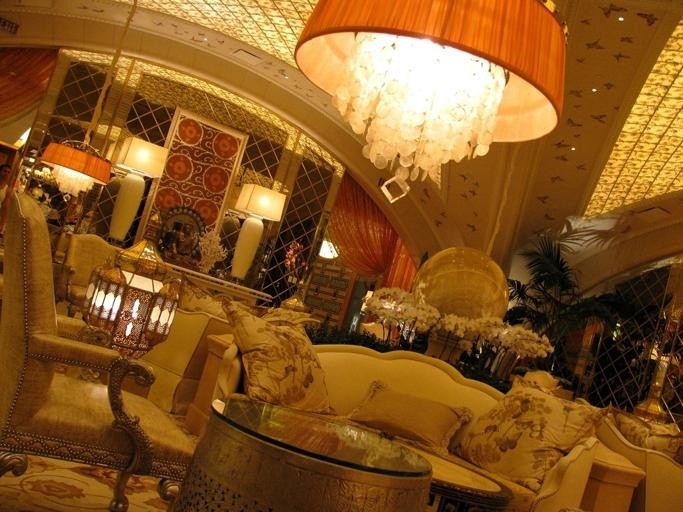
[424,331,524,384]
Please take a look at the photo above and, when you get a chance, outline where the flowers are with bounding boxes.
[363,286,555,360]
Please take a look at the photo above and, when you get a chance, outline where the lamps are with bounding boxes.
[38,0,172,242]
[231,183,288,279]
[294,0,566,204]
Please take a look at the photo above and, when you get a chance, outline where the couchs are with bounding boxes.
[182,330,647,512]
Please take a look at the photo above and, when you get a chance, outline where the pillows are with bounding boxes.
[610,406,682,459]
[223,302,331,415]
[346,377,600,494]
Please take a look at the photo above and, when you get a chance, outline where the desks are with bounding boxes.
[170,395,513,510]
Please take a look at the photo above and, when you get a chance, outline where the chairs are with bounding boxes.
[1,190,195,512]
